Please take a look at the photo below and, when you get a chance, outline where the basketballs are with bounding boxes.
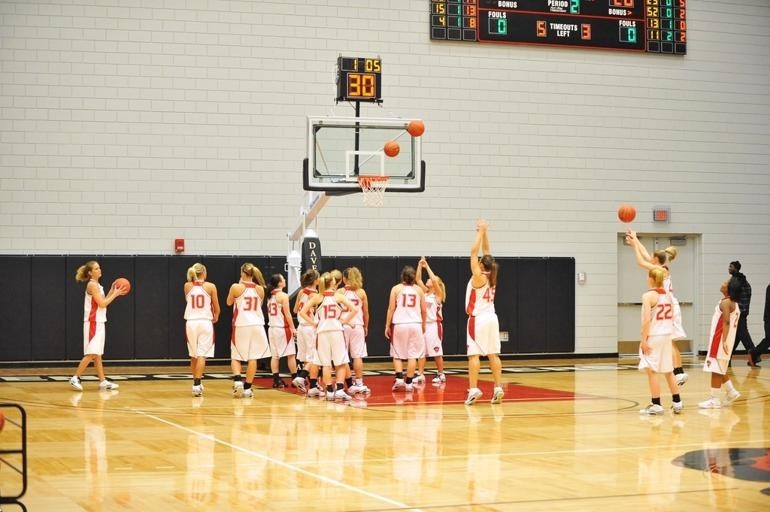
[0,412,5,432]
[409,121,425,137]
[114,278,130,295]
[619,206,636,221]
[385,141,400,156]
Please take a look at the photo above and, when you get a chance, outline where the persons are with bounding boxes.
[184,262,221,397]
[69,261,128,391]
[747,283,770,367]
[299,271,359,400]
[624,226,688,388]
[638,267,685,414]
[463,220,505,405]
[384,264,427,393]
[336,266,371,395]
[728,261,761,368]
[267,273,309,388]
[410,255,446,385]
[293,269,325,397]
[696,279,742,409]
[226,262,271,398]
[330,270,343,289]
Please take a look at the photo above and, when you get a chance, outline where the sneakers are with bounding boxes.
[244,388,254,398]
[69,376,83,391]
[491,387,504,404]
[748,349,757,367]
[326,390,335,401]
[99,378,119,390]
[670,400,682,414]
[192,384,204,397]
[405,382,414,391]
[748,356,761,366]
[273,379,288,388]
[675,373,687,386]
[233,381,244,399]
[292,376,307,393]
[432,373,446,383]
[392,378,405,392]
[722,388,740,405]
[347,382,371,394]
[335,388,352,402]
[463,388,483,405]
[307,382,325,397]
[697,398,721,409]
[412,371,425,383]
[639,402,665,415]
